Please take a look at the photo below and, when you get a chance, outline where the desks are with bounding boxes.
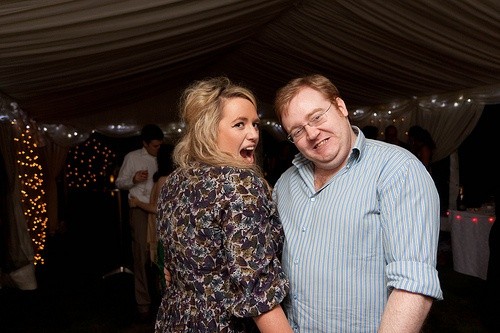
[448,208,496,280]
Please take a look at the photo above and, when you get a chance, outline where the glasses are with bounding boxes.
[287,102,333,144]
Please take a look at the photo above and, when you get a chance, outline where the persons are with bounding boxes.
[270,77,444,333]
[129,144,178,311]
[357,121,440,186]
[156,77,297,332]
[115,126,165,318]
